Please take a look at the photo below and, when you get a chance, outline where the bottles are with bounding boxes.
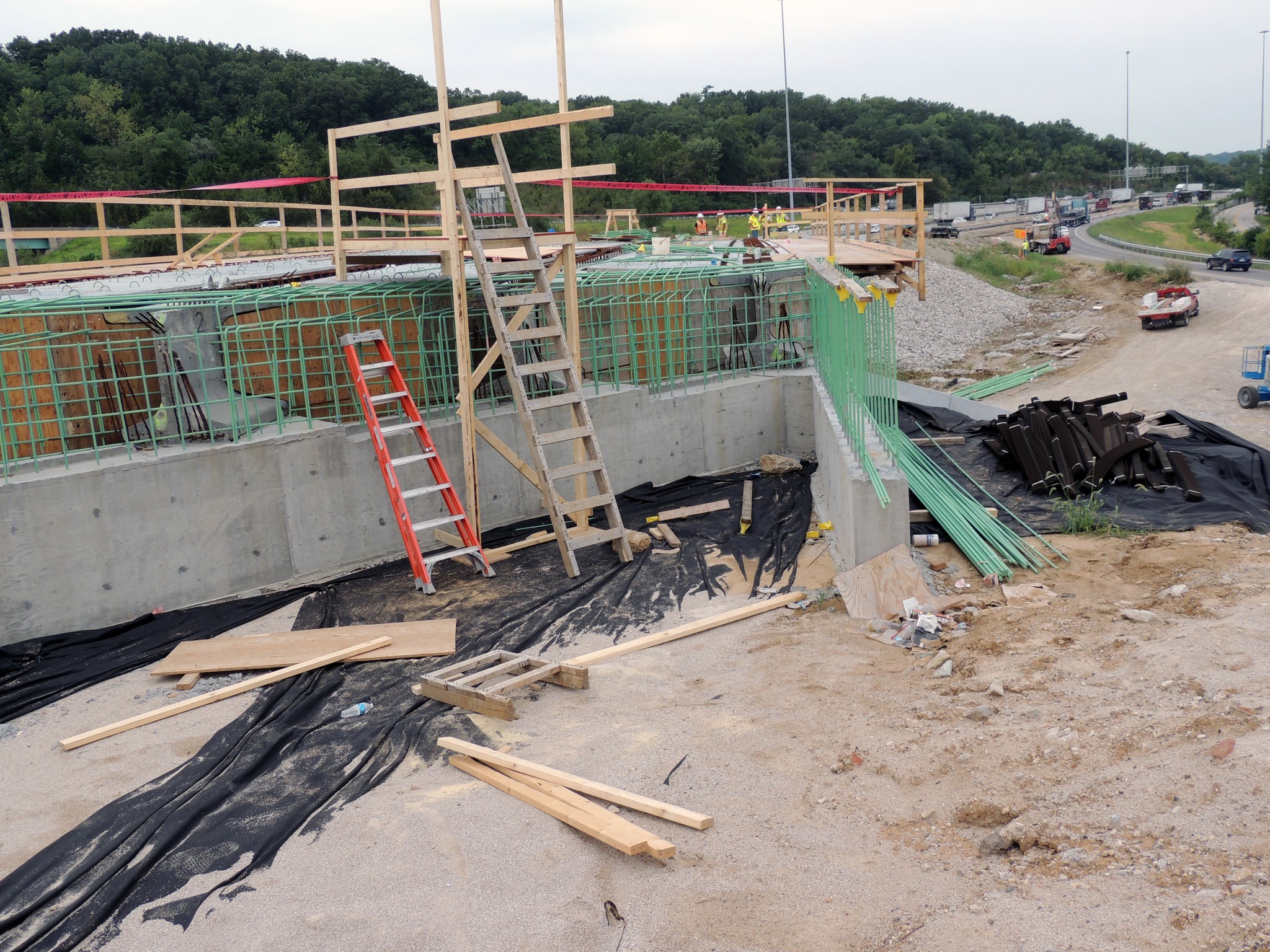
[340,702,373,718]
[637,244,645,255]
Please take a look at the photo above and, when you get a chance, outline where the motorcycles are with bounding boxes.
[1177,191,1193,204]
[1195,189,1212,201]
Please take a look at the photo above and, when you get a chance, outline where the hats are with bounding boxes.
[752,208,759,213]
[762,206,765,209]
[717,210,724,215]
[775,206,782,211]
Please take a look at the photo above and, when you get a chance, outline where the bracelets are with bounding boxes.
[707,230,708,232]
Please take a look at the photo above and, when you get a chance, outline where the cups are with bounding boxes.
[912,534,939,547]
[748,234,750,238]
[719,231,722,236]
[708,231,713,236]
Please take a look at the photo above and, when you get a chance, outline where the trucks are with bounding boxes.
[933,201,976,223]
[877,199,897,211]
[1137,287,1200,330]
[1093,197,1112,213]
[1032,212,1049,223]
[1138,195,1154,210]
[1174,183,1203,198]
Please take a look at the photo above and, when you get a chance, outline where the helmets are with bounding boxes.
[697,213,704,218]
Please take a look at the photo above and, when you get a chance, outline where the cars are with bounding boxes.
[984,212,995,219]
[952,217,967,223]
[1055,226,1069,236]
[1152,199,1164,207]
[1005,198,1015,204]
[834,206,850,212]
[787,225,800,234]
[253,220,280,228]
[870,207,879,212]
[1166,193,1173,199]
[1167,198,1178,205]
[870,224,880,233]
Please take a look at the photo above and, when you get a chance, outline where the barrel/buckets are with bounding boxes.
[652,237,670,259]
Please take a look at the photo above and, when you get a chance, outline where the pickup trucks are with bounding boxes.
[930,222,960,239]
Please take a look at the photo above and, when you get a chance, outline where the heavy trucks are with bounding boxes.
[1015,197,1049,215]
[1103,188,1136,204]
[1026,221,1071,255]
[1059,198,1091,227]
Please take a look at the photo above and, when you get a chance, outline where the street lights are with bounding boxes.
[1125,50,1131,188]
[1258,31,1269,180]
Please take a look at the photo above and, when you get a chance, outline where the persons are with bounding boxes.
[776,206,790,231]
[759,207,773,239]
[715,210,728,235]
[748,208,759,238]
[1022,241,1028,255]
[695,213,708,235]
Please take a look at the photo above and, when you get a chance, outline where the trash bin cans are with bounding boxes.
[1014,227,1018,238]
[1017,227,1026,239]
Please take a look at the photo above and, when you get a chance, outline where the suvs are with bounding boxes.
[1205,248,1253,272]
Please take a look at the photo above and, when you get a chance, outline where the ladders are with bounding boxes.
[438,133,633,577]
[340,329,496,595]
